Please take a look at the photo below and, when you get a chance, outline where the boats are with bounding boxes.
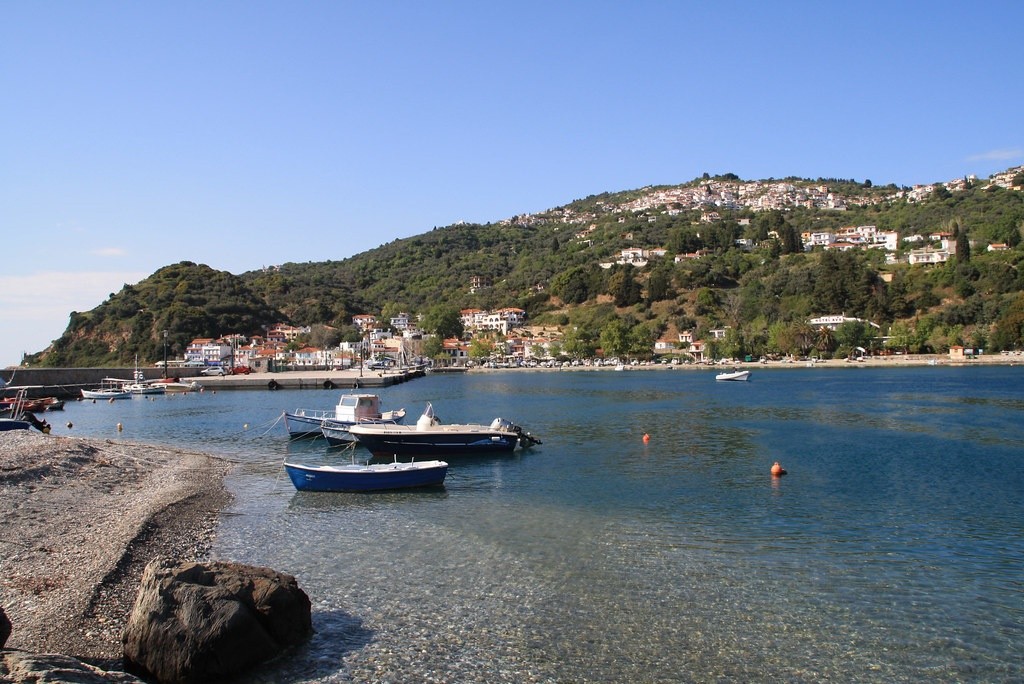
[283,453,448,492]
[0,388,66,432]
[348,400,543,454]
[281,392,407,437]
[126,355,196,394]
[716,370,753,382]
[321,393,398,446]
[80,388,134,399]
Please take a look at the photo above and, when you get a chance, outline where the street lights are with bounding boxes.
[273,340,279,373]
[163,330,169,379]
[360,342,364,377]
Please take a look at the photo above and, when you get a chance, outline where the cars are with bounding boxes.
[230,364,251,375]
[200,366,230,376]
[368,362,391,371]
